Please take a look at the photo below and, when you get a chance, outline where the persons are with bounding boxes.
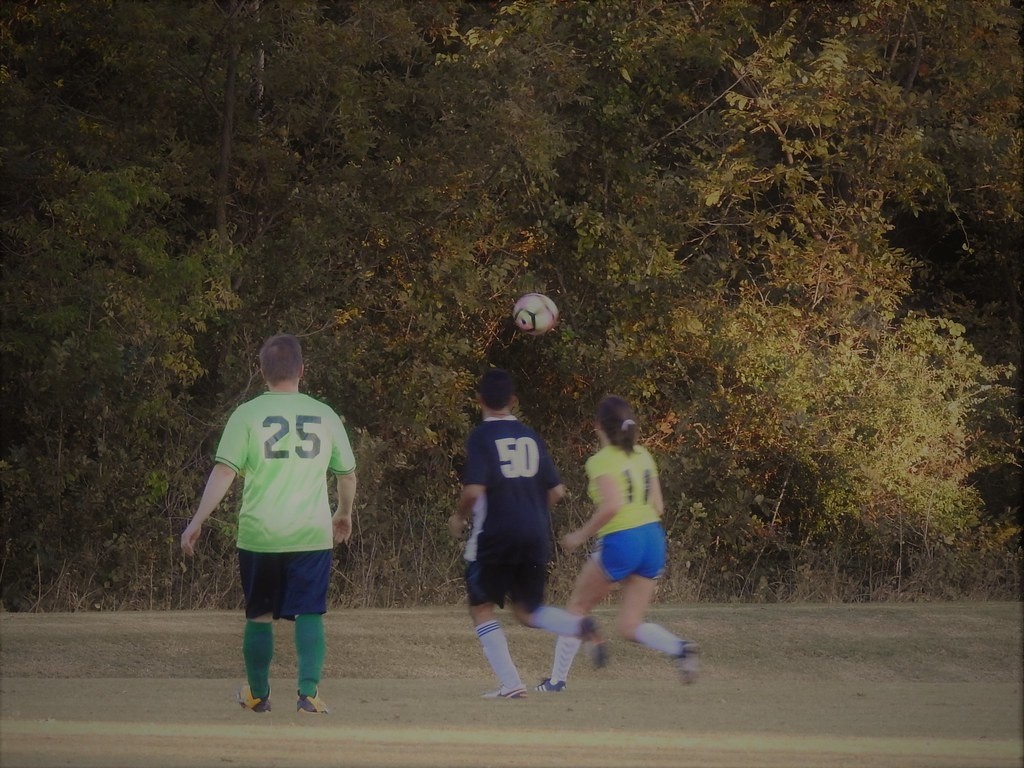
[178,335,359,714]
[447,369,609,700]
[535,394,699,692]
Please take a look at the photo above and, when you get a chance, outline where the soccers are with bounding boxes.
[512,293,559,337]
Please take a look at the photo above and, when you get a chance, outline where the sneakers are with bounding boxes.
[534,681,567,696]
[240,685,273,714]
[296,686,329,717]
[679,641,699,686]
[486,685,531,700]
[582,615,610,669]
